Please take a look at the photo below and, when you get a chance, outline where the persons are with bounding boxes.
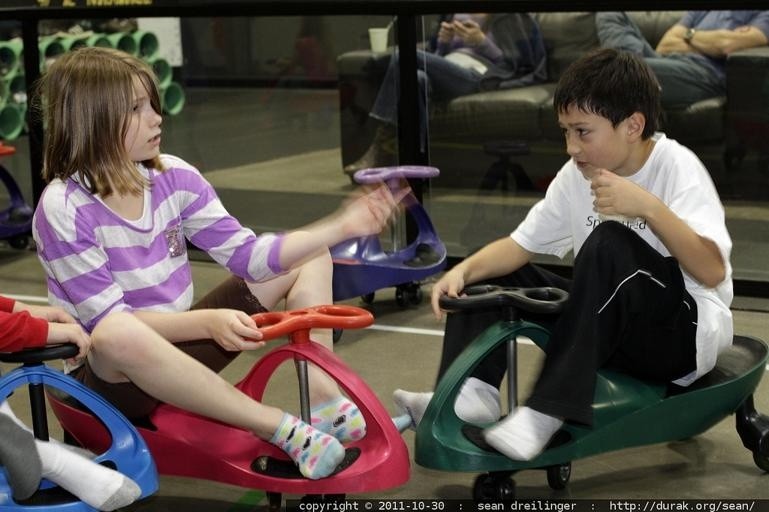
[32,46,412,480]
[342,12,548,178]
[1,294,143,510]
[392,47,736,462]
[601,6,769,107]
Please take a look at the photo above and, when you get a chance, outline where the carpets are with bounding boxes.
[202,147,769,221]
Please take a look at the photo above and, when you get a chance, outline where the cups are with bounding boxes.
[368,28,389,53]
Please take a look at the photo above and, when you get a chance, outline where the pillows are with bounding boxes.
[526,11,687,81]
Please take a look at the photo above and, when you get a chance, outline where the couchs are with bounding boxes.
[336,10,769,199]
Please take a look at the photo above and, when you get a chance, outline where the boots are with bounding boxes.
[345,128,400,176]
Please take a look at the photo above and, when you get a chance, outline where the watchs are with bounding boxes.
[681,28,697,43]
